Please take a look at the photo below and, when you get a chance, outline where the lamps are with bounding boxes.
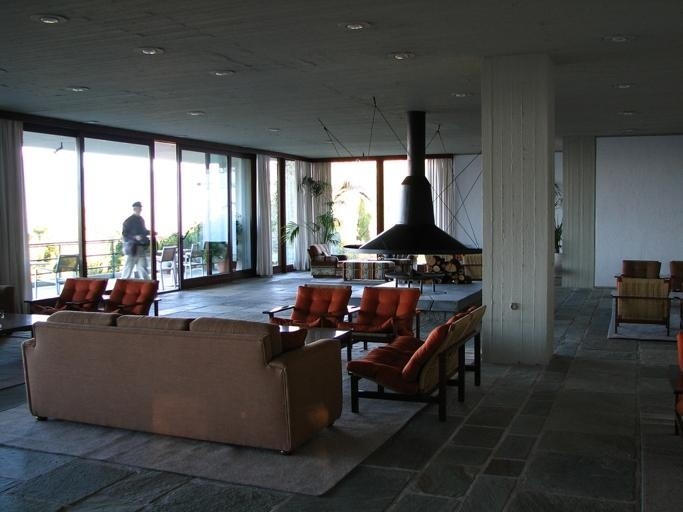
[359,109,474,257]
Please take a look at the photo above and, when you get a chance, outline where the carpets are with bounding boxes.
[608,290,683,341]
[0,356,426,494]
[0,330,32,390]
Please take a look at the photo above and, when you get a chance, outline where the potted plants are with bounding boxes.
[203,244,231,274]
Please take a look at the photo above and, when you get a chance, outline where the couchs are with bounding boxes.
[307,243,347,279]
[615,259,666,284]
[668,261,683,293]
[21,310,345,453]
[612,276,673,335]
[342,261,396,282]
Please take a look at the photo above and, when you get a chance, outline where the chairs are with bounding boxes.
[183,242,204,280]
[349,313,471,421]
[152,245,179,289]
[262,280,352,330]
[25,277,106,318]
[380,302,487,404]
[345,286,420,359]
[55,277,163,315]
[52,253,80,294]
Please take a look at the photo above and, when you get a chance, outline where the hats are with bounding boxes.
[132,201,143,207]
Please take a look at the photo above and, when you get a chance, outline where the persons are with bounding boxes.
[122,201,157,279]
